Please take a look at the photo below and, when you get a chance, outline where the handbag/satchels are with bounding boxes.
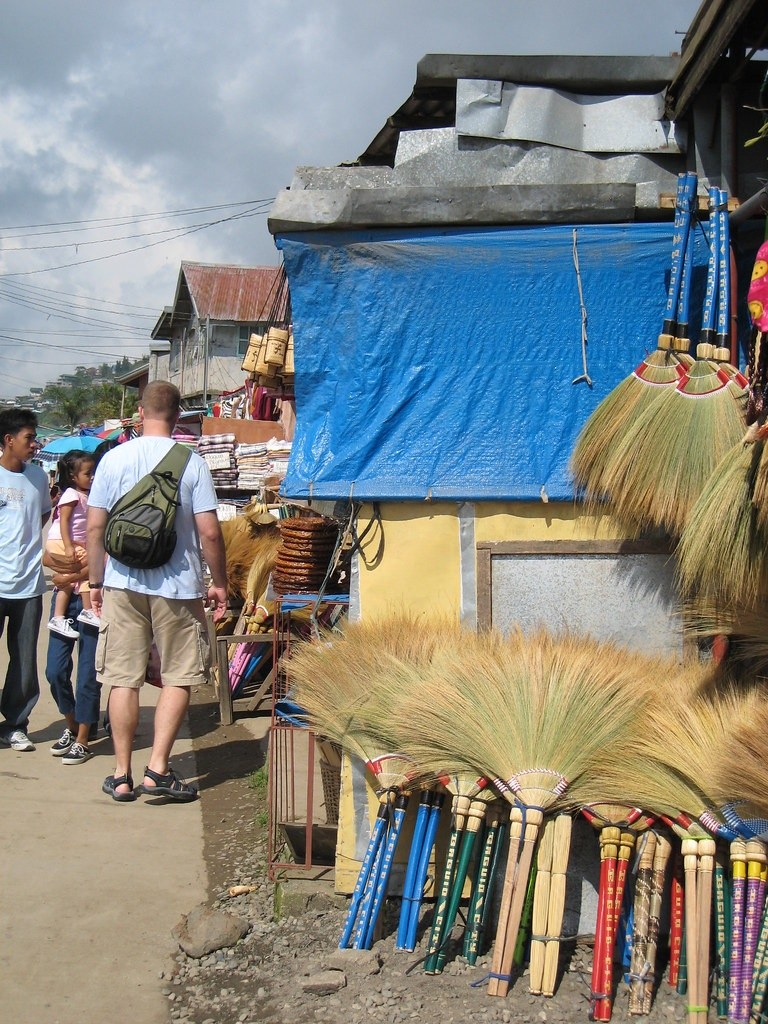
[104,443,193,569]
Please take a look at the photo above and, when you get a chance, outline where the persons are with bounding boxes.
[86,380,228,800]
[0,408,53,750]
[42,440,121,765]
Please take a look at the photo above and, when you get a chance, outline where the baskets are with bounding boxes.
[320,758,342,826]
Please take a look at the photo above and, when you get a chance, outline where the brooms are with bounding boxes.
[203,165,768,1023]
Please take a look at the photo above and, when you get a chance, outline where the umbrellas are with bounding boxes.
[34,437,104,461]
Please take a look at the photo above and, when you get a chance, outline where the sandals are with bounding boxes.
[143,766,197,797]
[102,773,137,801]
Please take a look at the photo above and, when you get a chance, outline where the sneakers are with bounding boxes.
[62,742,94,765]
[77,608,101,628]
[47,616,80,638]
[0,729,34,751]
[50,728,76,756]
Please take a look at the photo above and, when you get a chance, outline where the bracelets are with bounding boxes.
[89,580,104,588]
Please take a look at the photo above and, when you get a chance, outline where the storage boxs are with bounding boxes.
[267,730,341,824]
[204,399,294,442]
